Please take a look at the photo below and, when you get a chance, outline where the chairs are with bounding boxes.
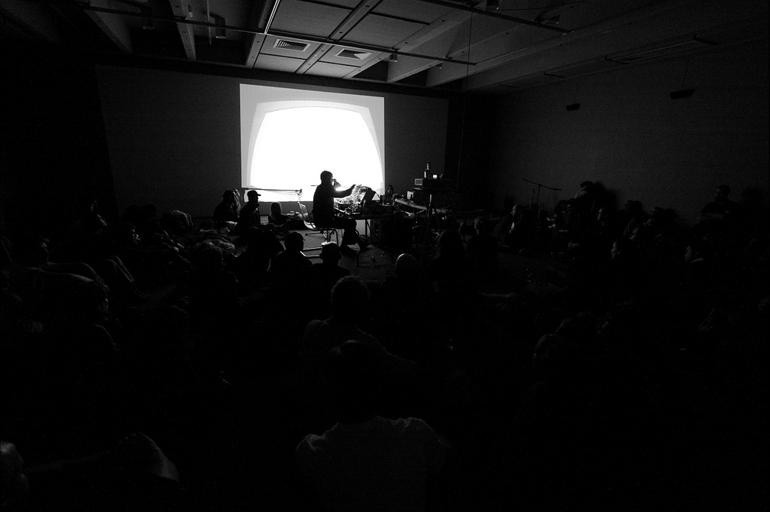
[298,202,341,259]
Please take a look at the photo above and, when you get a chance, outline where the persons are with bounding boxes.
[2,185,770,505]
[313,172,357,245]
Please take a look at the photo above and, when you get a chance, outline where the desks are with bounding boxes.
[335,203,391,254]
[393,199,458,217]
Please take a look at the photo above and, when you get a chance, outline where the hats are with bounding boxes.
[248,191,261,197]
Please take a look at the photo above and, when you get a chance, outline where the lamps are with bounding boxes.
[384,52,402,65]
[210,24,230,39]
